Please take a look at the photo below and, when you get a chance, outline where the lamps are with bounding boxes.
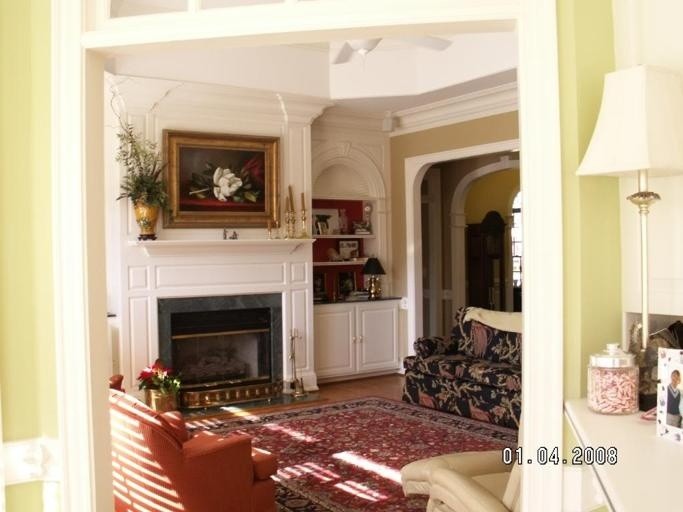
[360,258,386,301]
[575,64,682,411]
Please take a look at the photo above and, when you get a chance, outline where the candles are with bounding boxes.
[285,185,306,211]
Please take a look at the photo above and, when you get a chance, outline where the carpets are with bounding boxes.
[184,396,518,512]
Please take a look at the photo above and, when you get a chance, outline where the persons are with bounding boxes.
[313,217,328,234]
[313,274,322,293]
[666,369,680,427]
[340,272,353,292]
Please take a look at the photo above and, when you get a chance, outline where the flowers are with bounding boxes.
[136,358,181,395]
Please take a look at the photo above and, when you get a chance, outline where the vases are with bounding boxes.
[144,387,178,412]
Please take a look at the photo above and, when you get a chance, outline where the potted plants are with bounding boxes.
[110,76,173,240]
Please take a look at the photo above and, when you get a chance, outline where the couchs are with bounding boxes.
[401,307,522,430]
[109,374,277,512]
[398,450,518,512]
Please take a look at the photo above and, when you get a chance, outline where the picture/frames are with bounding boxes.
[657,348,683,442]
[336,269,357,300]
[162,129,281,230]
[337,240,360,261]
[313,272,327,298]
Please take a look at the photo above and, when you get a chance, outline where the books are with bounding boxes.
[313,295,329,305]
[342,289,369,301]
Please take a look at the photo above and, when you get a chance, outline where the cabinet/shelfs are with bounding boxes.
[312,194,378,267]
[314,300,399,383]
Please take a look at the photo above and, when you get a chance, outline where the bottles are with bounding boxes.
[587,340,641,415]
